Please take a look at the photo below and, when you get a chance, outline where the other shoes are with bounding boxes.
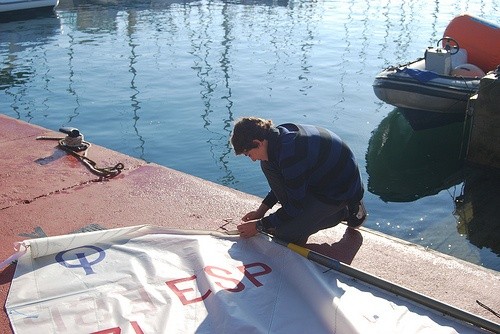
[347,201,367,229]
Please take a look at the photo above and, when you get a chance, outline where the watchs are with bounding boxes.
[255,218,265,233]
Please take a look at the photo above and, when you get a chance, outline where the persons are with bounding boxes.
[230,116,369,247]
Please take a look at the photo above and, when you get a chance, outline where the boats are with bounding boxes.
[0,0,60,13]
[372,35,482,116]
[366,106,470,203]
